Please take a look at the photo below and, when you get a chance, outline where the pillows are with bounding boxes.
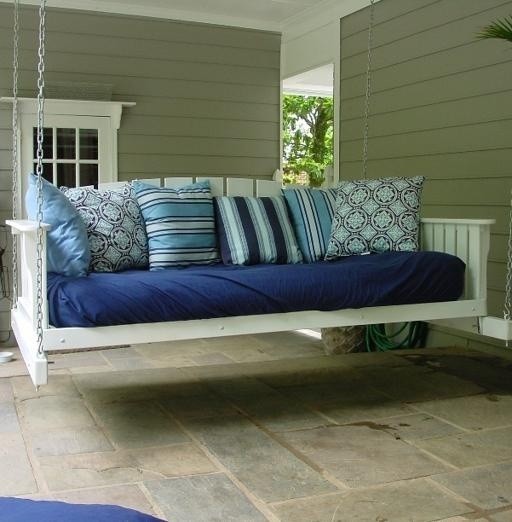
[282,188,338,262]
[64,184,149,272]
[131,179,221,270]
[25,172,91,278]
[325,176,424,261]
[214,196,303,265]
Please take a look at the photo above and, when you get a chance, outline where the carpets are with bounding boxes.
[1,498,164,521]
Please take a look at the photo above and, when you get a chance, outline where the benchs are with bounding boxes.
[6,176,512,392]
